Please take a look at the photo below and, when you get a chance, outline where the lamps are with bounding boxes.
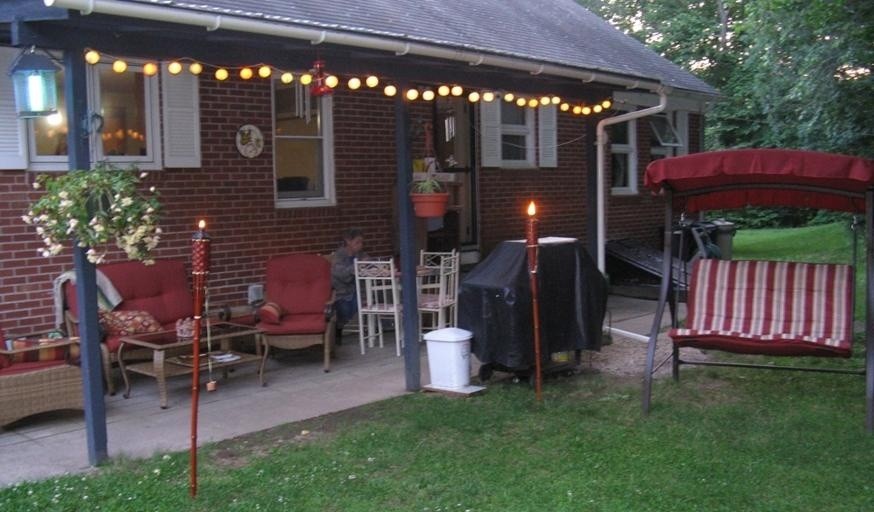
[188,219,213,492]
[521,198,548,406]
[3,38,68,122]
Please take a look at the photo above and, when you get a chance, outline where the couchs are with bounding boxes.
[253,247,346,376]
[0,323,106,436]
[47,257,256,390]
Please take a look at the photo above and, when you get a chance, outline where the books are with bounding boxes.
[162,349,240,367]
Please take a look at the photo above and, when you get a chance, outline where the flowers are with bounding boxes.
[22,156,172,269]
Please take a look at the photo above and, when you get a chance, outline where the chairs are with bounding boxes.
[353,243,462,357]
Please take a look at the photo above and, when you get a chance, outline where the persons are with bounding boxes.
[321,227,372,341]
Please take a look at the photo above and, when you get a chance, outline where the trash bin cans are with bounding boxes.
[422,327,473,389]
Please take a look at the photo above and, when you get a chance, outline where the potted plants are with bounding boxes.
[410,167,454,221]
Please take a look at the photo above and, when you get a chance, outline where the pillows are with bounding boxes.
[109,309,168,338]
[11,337,81,366]
[95,307,132,336]
